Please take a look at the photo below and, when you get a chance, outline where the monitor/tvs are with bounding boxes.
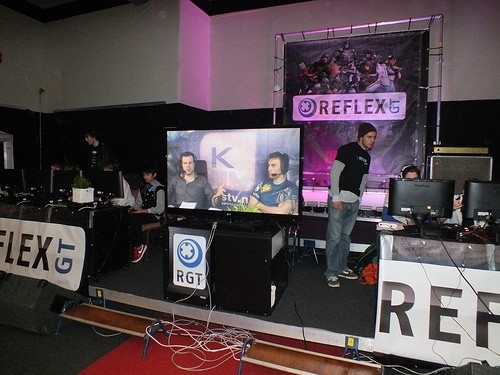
[83,169,124,199]
[463,179,500,227]
[162,124,303,232]
[0,168,27,195]
[388,178,455,227]
[50,170,82,193]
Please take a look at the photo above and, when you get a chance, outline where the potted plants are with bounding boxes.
[71,175,94,203]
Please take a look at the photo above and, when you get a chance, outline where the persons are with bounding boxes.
[82,129,110,188]
[126,166,165,263]
[441,178,489,227]
[297,38,402,95]
[94,160,135,214]
[324,123,377,287]
[248,151,299,214]
[167,152,226,208]
[383,165,463,225]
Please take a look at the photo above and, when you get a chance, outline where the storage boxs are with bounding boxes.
[426,156,493,195]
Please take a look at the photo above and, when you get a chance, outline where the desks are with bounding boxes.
[0,195,129,281]
[162,212,290,318]
[375,229,500,360]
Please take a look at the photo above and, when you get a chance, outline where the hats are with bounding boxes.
[358,122,376,139]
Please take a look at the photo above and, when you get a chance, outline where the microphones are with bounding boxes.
[272,168,289,176]
[177,165,186,172]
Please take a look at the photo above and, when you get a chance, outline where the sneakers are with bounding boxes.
[338,269,358,279]
[327,276,340,287]
[132,244,147,263]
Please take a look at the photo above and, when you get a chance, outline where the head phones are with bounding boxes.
[398,165,422,179]
[277,150,289,174]
[177,151,198,169]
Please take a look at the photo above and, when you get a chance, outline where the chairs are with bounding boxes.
[142,214,167,257]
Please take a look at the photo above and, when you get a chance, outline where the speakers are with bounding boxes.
[0,270,89,335]
